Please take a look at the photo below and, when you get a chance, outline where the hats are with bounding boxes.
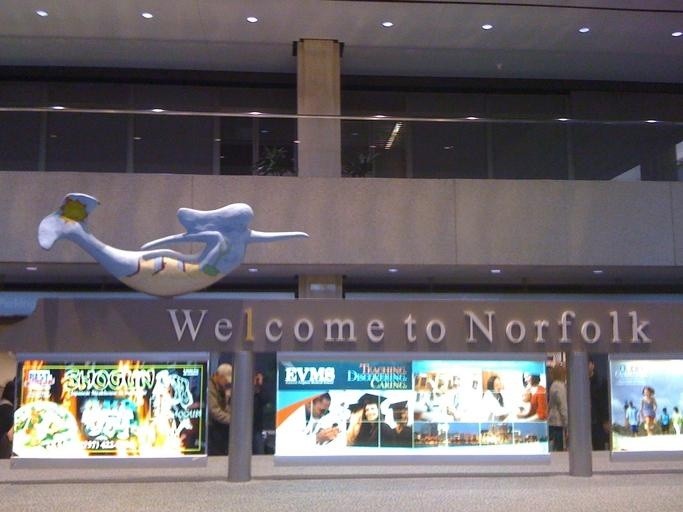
[357,393,385,407]
[389,400,409,410]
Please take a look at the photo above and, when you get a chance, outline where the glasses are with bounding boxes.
[317,405,330,416]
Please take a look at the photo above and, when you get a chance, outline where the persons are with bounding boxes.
[278,393,342,453]
[546,362,573,450]
[621,382,682,436]
[206,362,233,457]
[252,363,269,455]
[588,354,611,448]
[345,365,549,449]
